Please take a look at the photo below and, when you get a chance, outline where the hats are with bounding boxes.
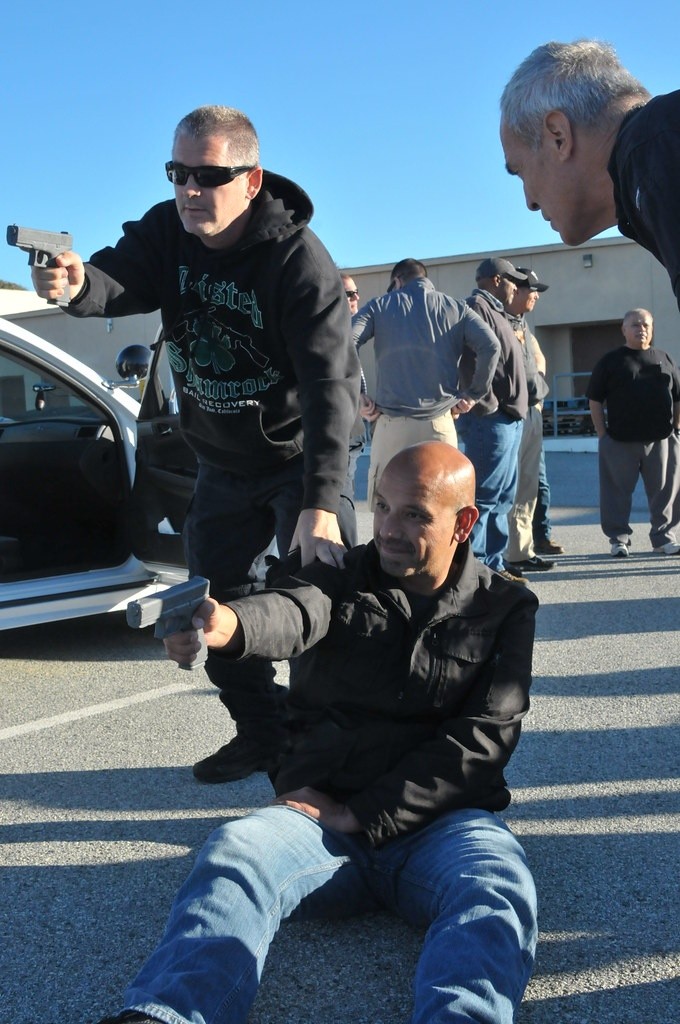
[475,257,528,282]
[516,266,550,292]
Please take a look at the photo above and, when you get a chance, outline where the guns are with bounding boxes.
[7,223,72,305]
[127,574,212,671]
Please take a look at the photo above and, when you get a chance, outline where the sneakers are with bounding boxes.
[508,554,557,571]
[505,565,522,576]
[611,543,630,557]
[533,542,562,554]
[653,539,680,554]
[498,570,529,586]
[192,728,270,783]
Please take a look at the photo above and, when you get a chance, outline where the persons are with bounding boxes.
[351,258,500,513]
[533,337,564,554]
[507,268,556,577]
[455,258,529,586]
[97,441,539,1024]
[499,41,680,313]
[586,309,680,556]
[340,273,367,479]
[32,105,360,782]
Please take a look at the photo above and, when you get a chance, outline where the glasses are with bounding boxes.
[387,273,404,293]
[164,159,255,188]
[346,288,359,297]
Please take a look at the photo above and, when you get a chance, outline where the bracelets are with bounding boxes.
[674,429,680,435]
